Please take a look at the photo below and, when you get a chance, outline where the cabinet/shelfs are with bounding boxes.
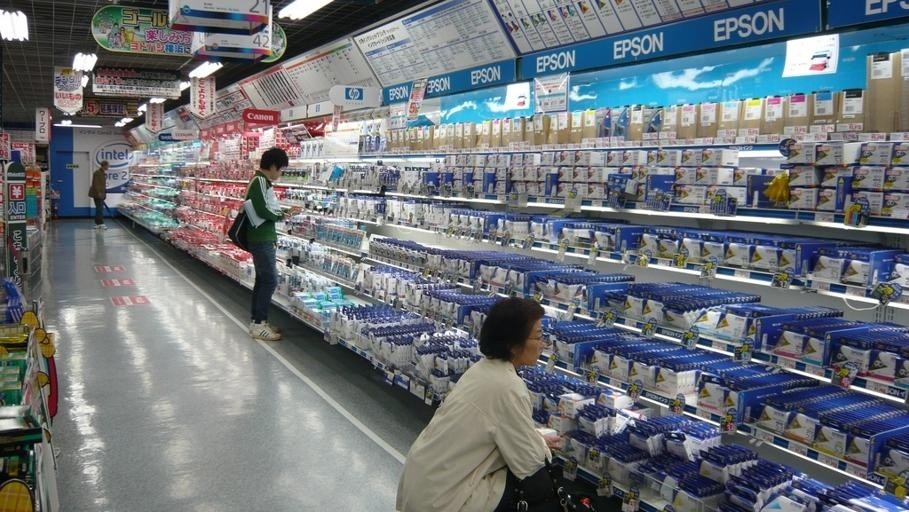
[242,181,907,509]
[174,177,249,284]
[118,162,179,235]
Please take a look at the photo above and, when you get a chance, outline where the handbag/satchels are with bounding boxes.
[560,492,600,512]
[228,212,247,252]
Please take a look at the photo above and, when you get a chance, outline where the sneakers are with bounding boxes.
[95,224,108,229]
[250,320,282,341]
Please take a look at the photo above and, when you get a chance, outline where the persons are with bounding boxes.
[237,149,288,341]
[88,160,109,230]
[396,297,561,512]
